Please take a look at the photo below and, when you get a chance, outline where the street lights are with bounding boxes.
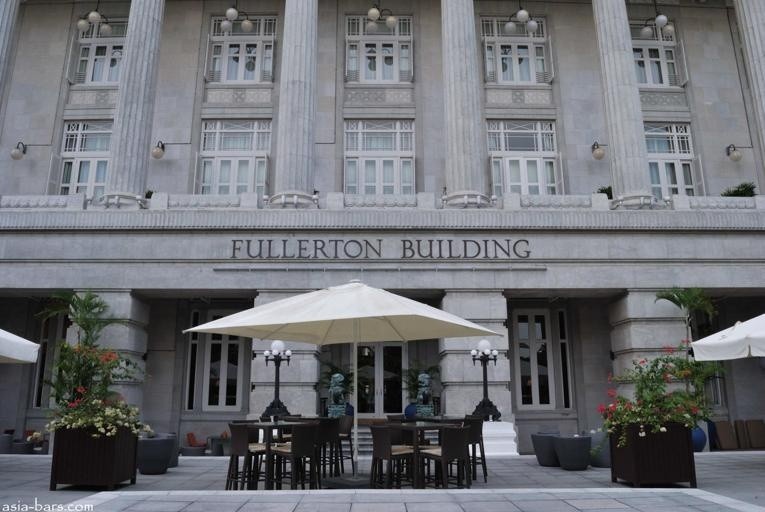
[470,338,504,422]
[260,338,292,424]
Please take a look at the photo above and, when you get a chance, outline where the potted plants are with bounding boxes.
[313,350,362,415]
[402,349,448,419]
[655,285,719,452]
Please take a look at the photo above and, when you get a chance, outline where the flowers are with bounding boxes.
[587,339,728,457]
[25,340,153,442]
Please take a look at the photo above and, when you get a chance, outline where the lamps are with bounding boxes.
[11,142,26,160]
[639,0,675,40]
[504,2,538,36]
[220,3,254,36]
[726,143,743,162]
[152,140,164,158]
[591,141,605,160]
[75,0,111,38]
[364,0,398,37]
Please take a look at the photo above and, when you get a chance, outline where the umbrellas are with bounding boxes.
[182,279,504,478]
[686,313,765,363]
[0,327,41,365]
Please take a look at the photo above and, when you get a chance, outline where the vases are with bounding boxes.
[531,432,591,470]
[137,432,178,475]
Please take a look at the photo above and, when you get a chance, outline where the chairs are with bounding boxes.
[224,414,355,490]
[370,415,488,490]
